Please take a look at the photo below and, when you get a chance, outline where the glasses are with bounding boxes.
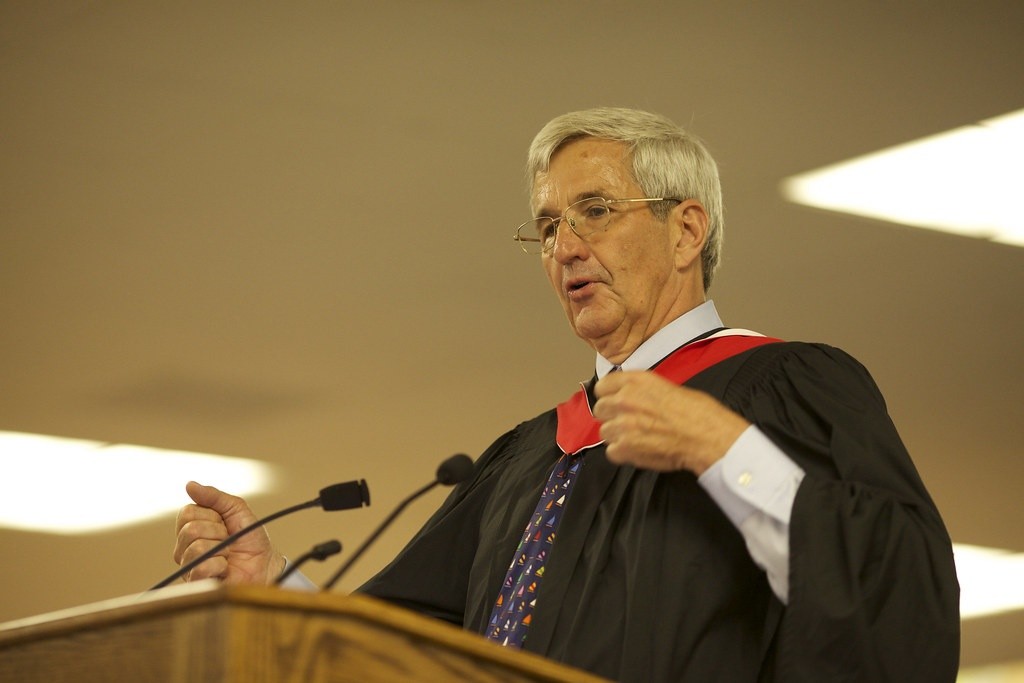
[513,197,681,253]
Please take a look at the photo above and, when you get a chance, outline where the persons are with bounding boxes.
[174,109,961,683]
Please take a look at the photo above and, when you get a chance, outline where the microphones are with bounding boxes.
[146,453,474,592]
